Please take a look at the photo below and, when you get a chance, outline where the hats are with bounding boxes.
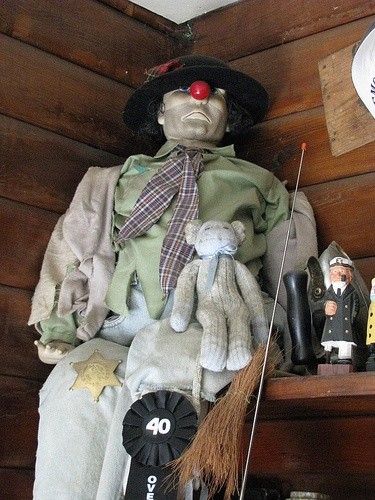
[123,54,270,139]
